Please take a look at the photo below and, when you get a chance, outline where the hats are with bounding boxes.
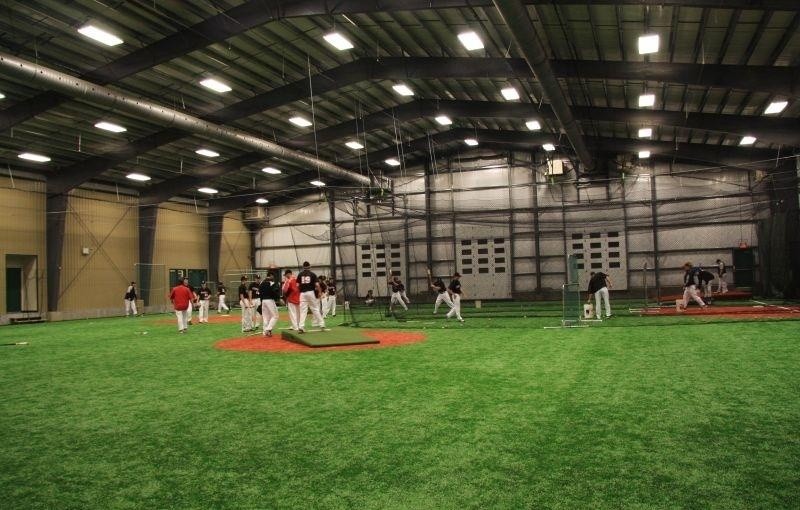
[240,276,249,279]
[303,262,310,267]
[283,269,292,276]
[184,277,189,279]
[201,280,205,283]
[131,282,136,284]
[255,275,260,278]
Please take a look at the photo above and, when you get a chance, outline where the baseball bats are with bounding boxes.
[428,269,433,286]
[389,269,392,282]
[1,342,28,346]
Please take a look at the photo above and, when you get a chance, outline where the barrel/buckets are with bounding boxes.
[48,310,63,321]
[583,303,593,320]
[474,300,482,308]
[675,298,686,312]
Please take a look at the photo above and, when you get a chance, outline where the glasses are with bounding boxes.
[454,272,462,277]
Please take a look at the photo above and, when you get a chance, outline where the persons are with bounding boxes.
[445,273,466,323]
[394,280,411,303]
[714,259,728,294]
[240,261,337,338]
[680,261,708,311]
[587,271,613,319]
[344,295,350,311]
[124,282,137,317]
[165,278,230,333]
[695,268,715,305]
[430,277,453,315]
[388,281,408,312]
[365,290,375,304]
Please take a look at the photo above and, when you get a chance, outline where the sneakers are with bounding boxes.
[446,314,465,322]
[242,327,325,337]
